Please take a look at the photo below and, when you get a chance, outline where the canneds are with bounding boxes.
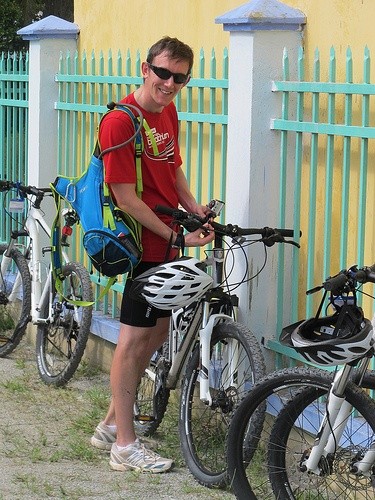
[116,232,138,258]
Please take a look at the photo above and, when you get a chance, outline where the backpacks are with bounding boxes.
[49,106,144,308]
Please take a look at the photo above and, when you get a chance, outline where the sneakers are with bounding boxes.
[92,423,158,451]
[109,442,175,473]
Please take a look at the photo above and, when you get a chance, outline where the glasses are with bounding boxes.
[145,61,188,85]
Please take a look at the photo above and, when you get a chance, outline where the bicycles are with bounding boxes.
[225,264,375,500]
[0,180,93,388]
[133,205,302,489]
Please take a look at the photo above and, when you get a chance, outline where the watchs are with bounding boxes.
[172,234,181,248]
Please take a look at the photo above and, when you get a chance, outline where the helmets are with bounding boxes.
[134,254,214,311]
[279,313,375,368]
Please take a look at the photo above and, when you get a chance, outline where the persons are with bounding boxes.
[91,39,215,473]
